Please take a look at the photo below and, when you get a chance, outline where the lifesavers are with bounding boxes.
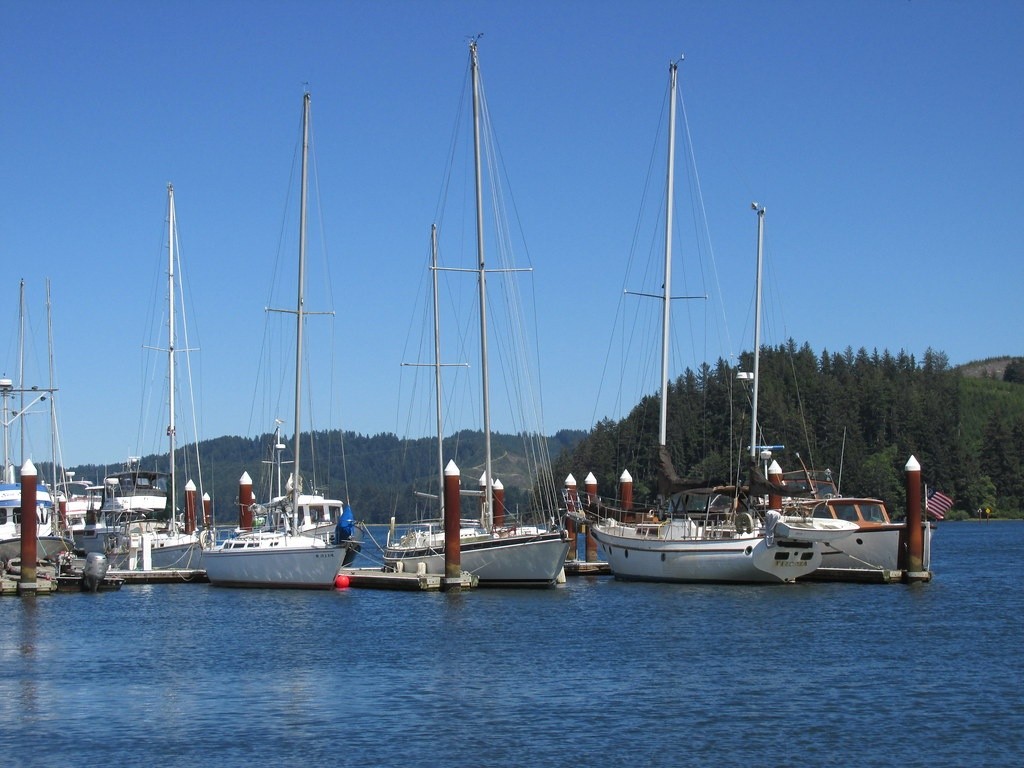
[200,530,216,551]
[733,513,753,535]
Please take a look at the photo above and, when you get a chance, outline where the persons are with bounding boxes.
[208,526,215,551]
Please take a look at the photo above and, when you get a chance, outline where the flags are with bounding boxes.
[925,484,953,521]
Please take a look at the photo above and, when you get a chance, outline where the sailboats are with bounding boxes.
[0,81,364,590]
[562,53,955,583]
[381,31,575,583]
